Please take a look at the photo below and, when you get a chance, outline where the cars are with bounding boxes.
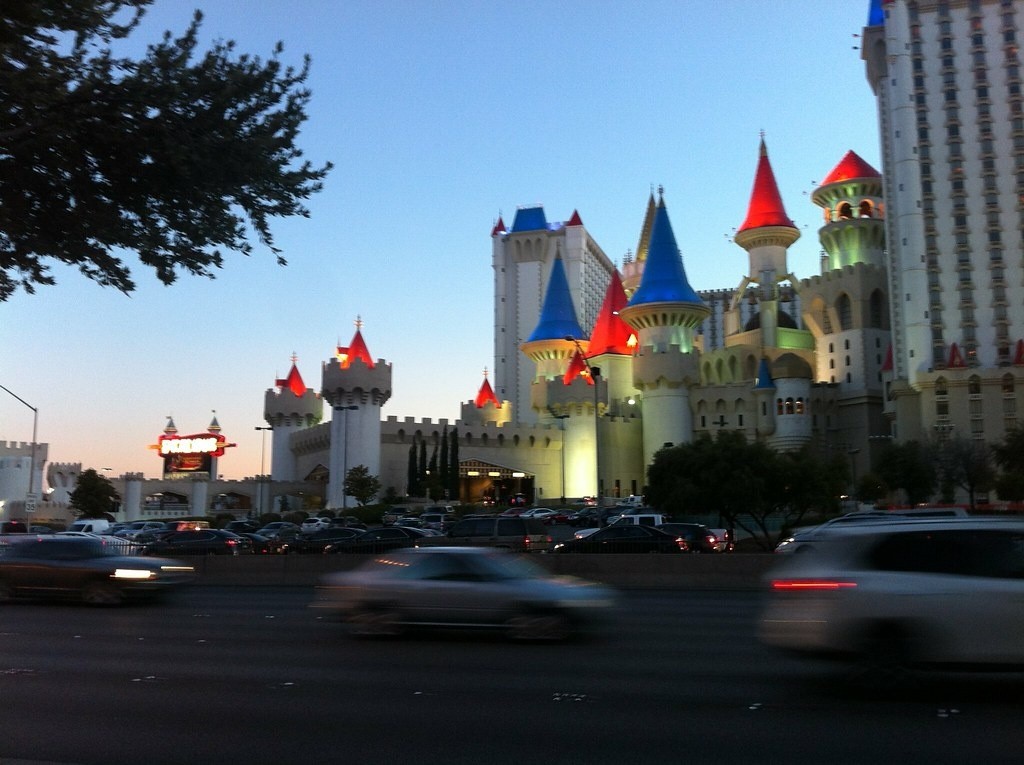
[0,533,188,605]
[0,494,732,554]
[314,545,623,641]
[741,515,1024,706]
[552,522,688,554]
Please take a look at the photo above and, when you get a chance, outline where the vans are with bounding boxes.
[776,506,969,558]
